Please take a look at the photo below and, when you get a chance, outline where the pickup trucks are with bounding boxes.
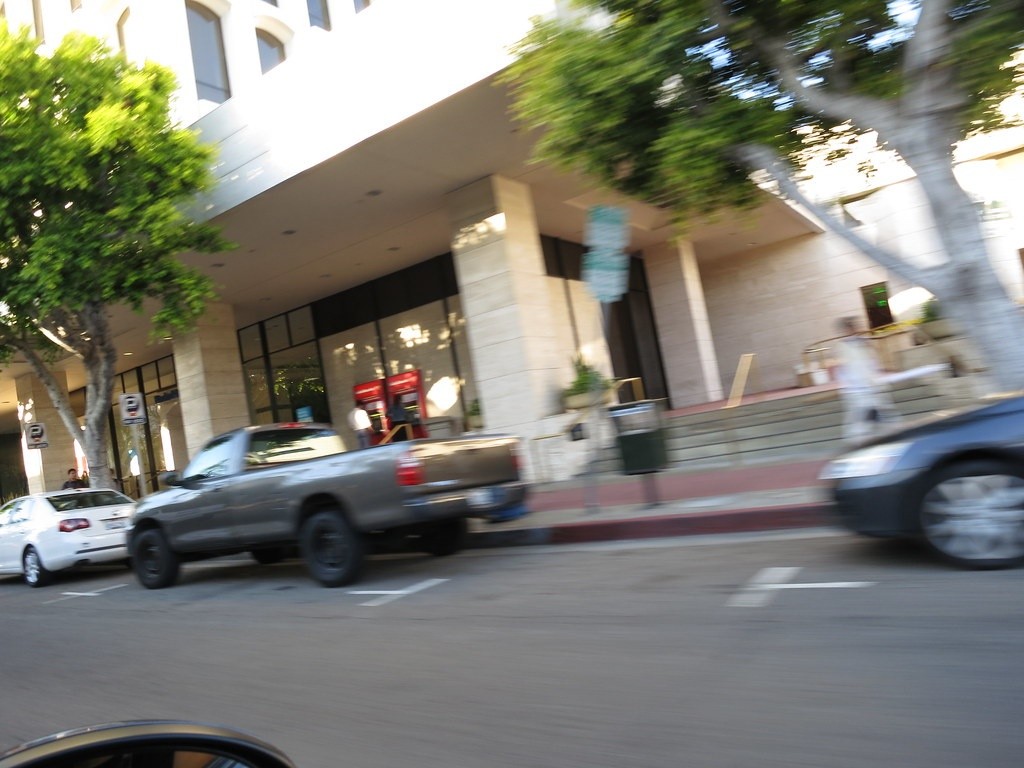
[124,421,537,590]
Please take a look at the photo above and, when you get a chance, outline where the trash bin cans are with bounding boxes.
[606,396,668,474]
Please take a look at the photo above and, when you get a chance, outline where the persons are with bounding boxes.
[347,399,375,448]
[384,393,415,440]
[60,469,123,493]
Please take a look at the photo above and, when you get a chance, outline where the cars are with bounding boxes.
[816,395,1024,572]
[0,487,137,587]
